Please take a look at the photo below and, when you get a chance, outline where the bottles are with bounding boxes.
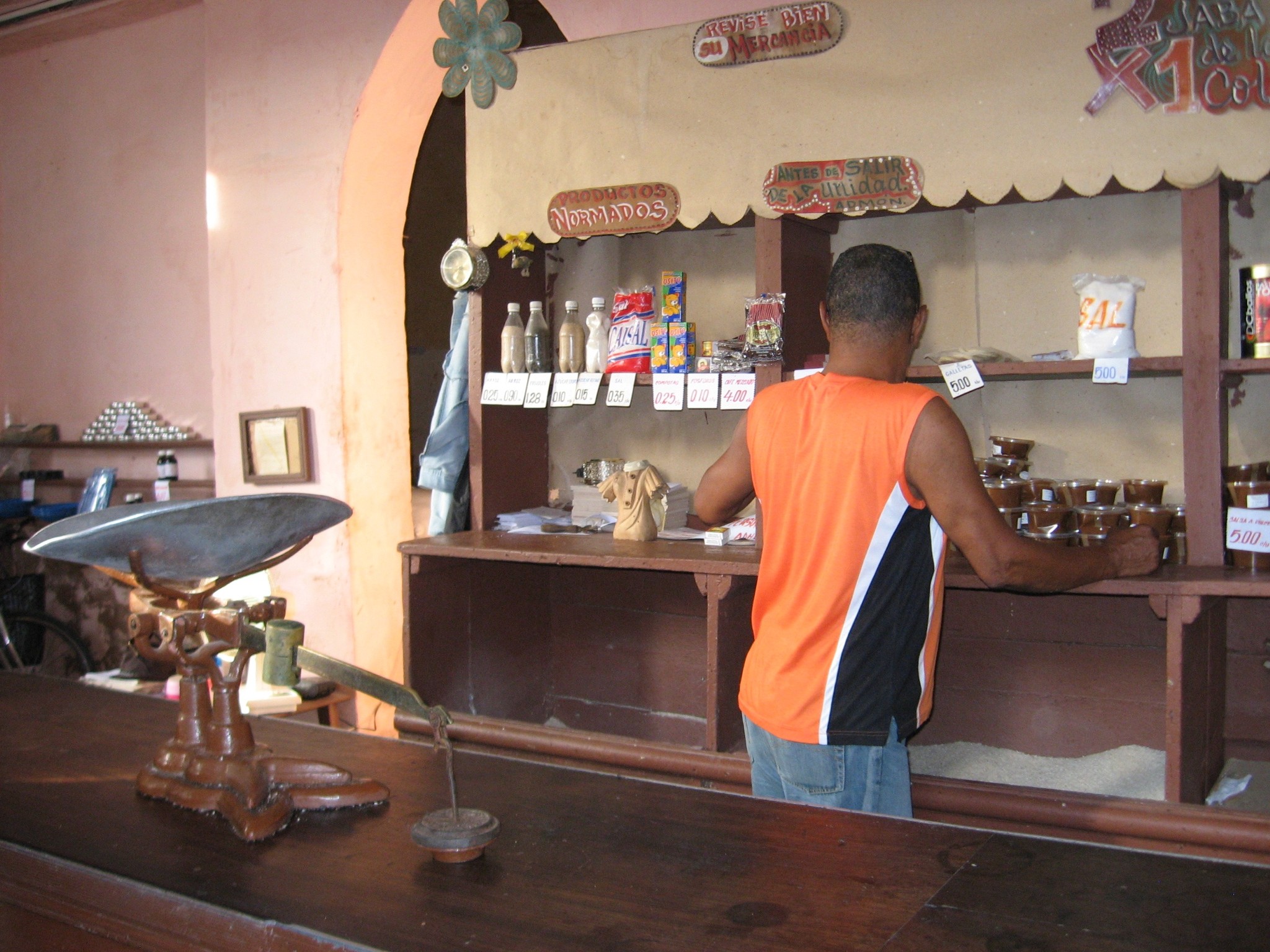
[126,495,135,504]
[165,450,179,479]
[558,300,584,373]
[524,301,551,373]
[135,493,143,503]
[156,451,166,480]
[500,303,526,372]
[585,297,610,373]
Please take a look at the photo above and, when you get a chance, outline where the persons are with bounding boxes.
[691,243,1172,823]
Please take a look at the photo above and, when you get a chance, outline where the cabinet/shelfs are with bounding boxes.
[2,434,213,525]
[398,174,1269,868]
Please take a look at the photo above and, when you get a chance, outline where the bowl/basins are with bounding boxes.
[1223,459,1269,570]
[974,436,1187,565]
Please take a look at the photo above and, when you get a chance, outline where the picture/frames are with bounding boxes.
[238,408,311,486]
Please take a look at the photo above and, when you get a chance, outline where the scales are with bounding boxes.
[20,490,500,868]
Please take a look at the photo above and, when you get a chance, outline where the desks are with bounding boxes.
[1,666,1269,951]
